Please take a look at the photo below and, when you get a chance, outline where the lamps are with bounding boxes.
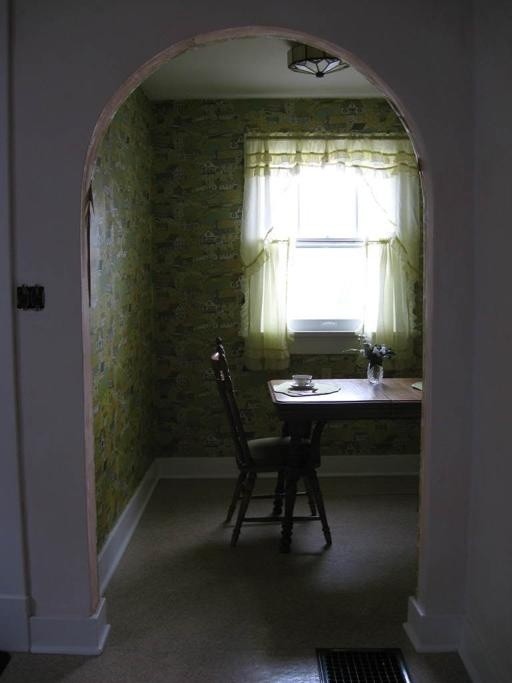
[287,44,349,78]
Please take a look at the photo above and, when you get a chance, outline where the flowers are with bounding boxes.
[342,325,396,369]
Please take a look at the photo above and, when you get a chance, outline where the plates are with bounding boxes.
[290,383,313,390]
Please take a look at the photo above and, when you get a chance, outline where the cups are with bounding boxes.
[293,374,312,386]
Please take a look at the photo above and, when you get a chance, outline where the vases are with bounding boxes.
[367,362,383,383]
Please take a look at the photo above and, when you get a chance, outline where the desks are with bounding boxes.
[268,376,422,553]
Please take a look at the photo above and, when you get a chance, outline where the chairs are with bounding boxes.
[209,337,332,547]
